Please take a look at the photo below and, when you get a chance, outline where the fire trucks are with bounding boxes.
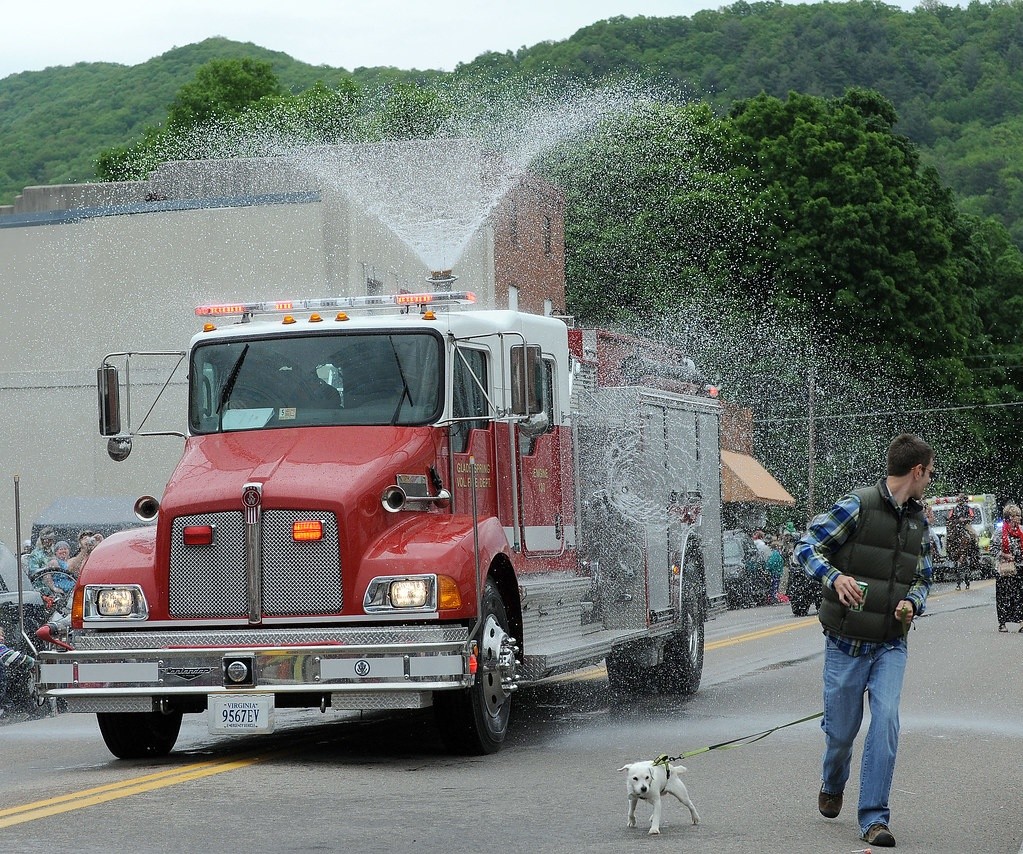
[924,492,1001,581]
[34,292,723,753]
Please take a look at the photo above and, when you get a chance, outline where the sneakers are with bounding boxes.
[861,827,896,846]
[817,785,844,819]
[998,626,1008,633]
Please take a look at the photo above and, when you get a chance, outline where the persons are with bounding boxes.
[940,493,982,560]
[989,505,1023,633]
[0,625,36,724]
[792,434,934,847]
[22,526,106,608]
[752,520,822,616]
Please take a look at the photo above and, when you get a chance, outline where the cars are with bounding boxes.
[718,529,767,603]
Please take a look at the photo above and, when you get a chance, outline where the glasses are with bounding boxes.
[911,466,936,478]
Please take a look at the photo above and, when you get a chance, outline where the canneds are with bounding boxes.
[847,582,869,614]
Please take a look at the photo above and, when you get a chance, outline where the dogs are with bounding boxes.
[617,761,700,833]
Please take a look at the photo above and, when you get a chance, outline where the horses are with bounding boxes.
[943,515,976,590]
